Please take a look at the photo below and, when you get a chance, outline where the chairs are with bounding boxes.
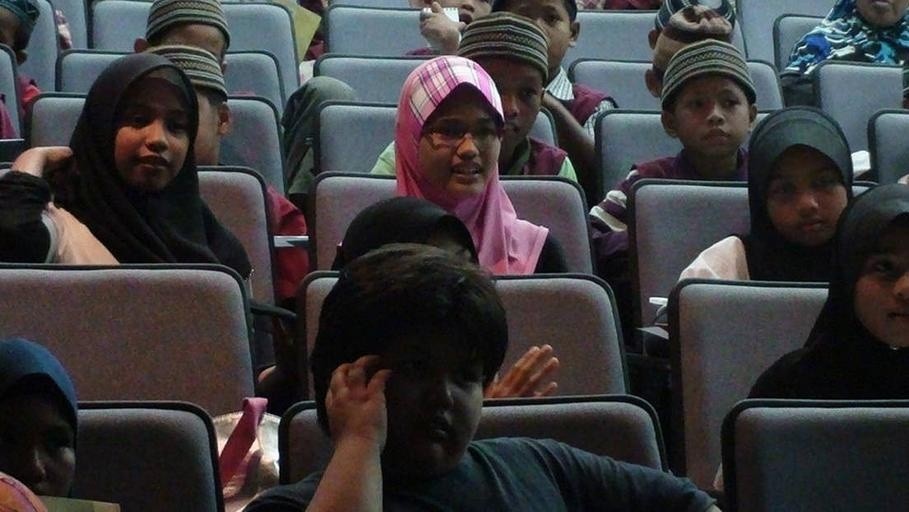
[1,0,909,512]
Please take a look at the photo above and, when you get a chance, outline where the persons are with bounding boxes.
[2,52,298,393]
[710,184,908,492]
[589,38,757,279]
[396,56,572,272]
[242,244,721,512]
[329,198,559,401]
[0,337,121,511]
[643,106,854,355]
[144,46,311,301]
[1,1,909,195]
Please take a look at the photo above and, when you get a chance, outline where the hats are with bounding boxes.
[150,48,227,98]
[147,1,230,48]
[1,1,40,29]
[649,0,755,110]
[459,14,549,78]
